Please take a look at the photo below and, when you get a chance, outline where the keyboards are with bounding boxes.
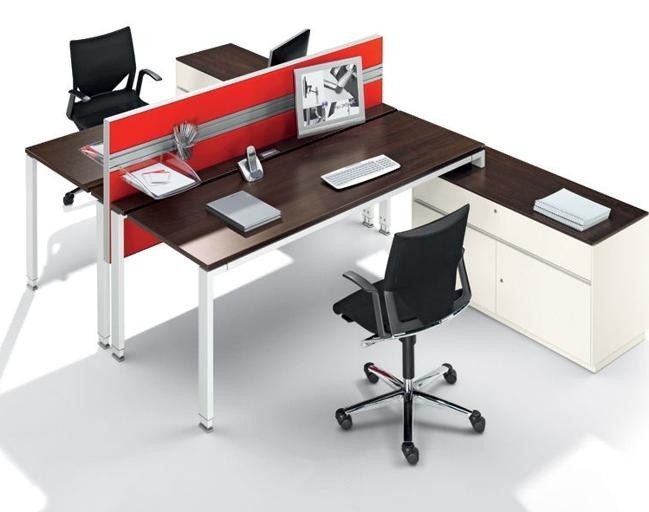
[320,153,402,190]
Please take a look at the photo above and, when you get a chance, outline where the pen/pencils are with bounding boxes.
[173,121,198,161]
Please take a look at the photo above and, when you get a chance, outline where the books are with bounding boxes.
[206,189,282,234]
[533,204,610,232]
[535,186,611,226]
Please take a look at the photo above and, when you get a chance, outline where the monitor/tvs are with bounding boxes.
[294,55,366,139]
[268,29,310,67]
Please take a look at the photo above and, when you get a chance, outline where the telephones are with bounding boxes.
[237,145,265,183]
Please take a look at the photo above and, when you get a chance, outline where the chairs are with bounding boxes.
[331,202,490,463]
[58,23,163,206]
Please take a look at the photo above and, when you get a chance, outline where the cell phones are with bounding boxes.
[246,145,257,173]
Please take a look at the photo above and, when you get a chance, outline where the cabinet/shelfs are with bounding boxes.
[416,179,649,369]
